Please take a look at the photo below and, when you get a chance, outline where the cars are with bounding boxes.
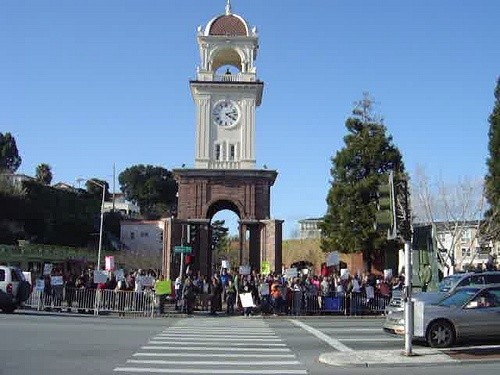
[383,284,500,349]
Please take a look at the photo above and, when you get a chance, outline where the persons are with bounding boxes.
[255,272,404,316]
[244,274,255,288]
[211,277,223,314]
[175,276,182,309]
[199,275,211,311]
[239,278,252,316]
[23,263,155,317]
[154,274,168,313]
[233,270,239,290]
[230,267,235,279]
[213,273,221,287]
[182,279,198,314]
[221,268,234,303]
[225,279,237,314]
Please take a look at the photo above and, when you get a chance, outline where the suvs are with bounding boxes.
[385,271,500,315]
[1,266,30,313]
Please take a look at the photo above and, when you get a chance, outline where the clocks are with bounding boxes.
[210,98,242,130]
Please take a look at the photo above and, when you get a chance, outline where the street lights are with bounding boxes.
[76,176,106,269]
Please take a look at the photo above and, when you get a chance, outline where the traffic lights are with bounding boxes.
[373,170,397,240]
